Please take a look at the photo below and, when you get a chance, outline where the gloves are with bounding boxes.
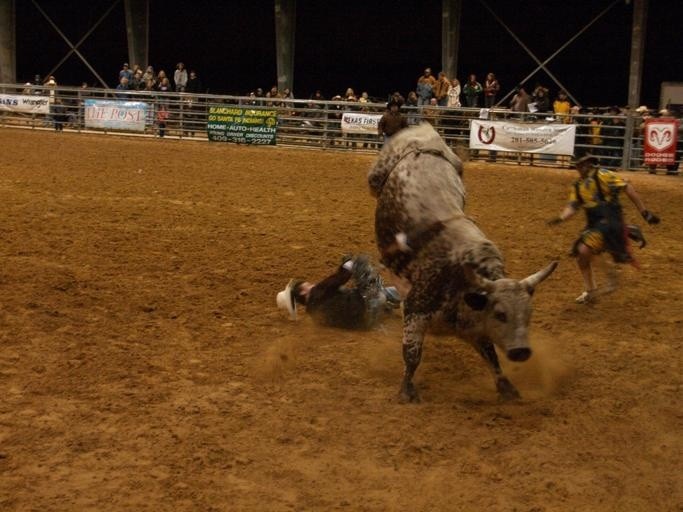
[642,210,659,224]
[546,216,562,226]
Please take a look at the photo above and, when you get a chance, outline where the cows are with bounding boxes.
[367,120,560,404]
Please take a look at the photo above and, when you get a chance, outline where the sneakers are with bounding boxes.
[575,287,599,304]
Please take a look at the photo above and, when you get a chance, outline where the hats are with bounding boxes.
[276,278,297,318]
[331,88,367,102]
[635,105,649,112]
[569,151,599,165]
[47,80,56,85]
[48,76,54,79]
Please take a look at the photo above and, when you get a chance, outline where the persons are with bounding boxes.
[116,63,202,137]
[291,254,402,333]
[386,69,679,176]
[246,87,296,116]
[23,75,67,131]
[543,151,659,303]
[300,88,384,148]
[80,83,90,116]
